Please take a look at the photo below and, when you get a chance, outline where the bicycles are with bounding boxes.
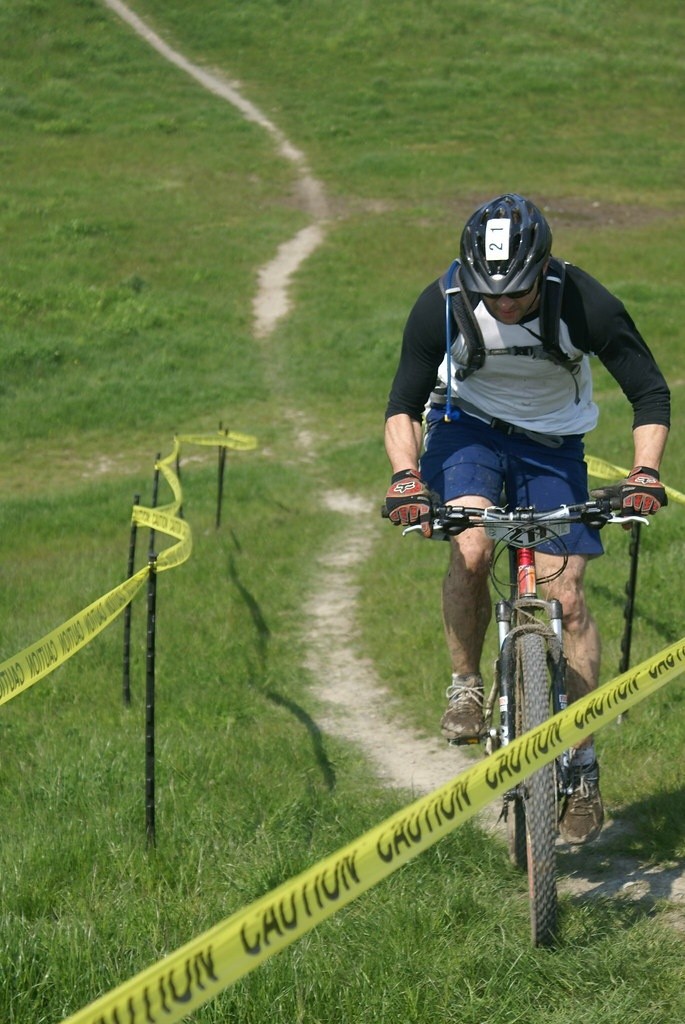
[384,485,648,947]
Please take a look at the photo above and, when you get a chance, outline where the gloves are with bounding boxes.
[590,466,668,530]
[386,469,433,538]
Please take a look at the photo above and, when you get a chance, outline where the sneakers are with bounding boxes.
[559,761,604,846]
[439,678,488,739]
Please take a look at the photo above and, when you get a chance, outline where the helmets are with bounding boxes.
[460,192,553,294]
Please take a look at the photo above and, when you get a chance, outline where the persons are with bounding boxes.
[380,191,673,849]
